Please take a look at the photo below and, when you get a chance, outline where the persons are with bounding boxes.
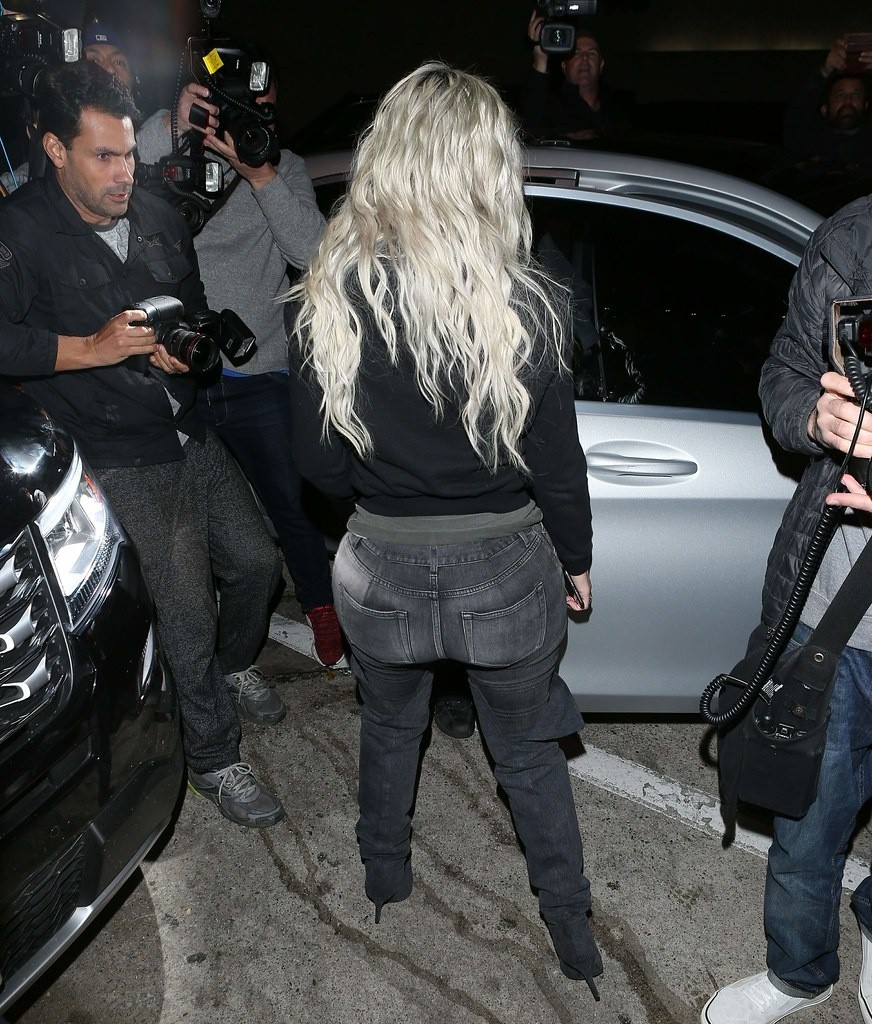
[308,62,605,1004]
[515,0,616,202]
[0,61,289,832]
[130,36,346,666]
[701,194,871,1024]
[802,29,872,218]
[0,0,135,196]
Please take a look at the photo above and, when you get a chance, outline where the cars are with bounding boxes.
[285,148,825,714]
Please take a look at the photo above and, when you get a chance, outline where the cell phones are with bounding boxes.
[841,32,872,73]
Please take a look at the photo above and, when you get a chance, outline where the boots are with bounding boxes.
[349,653,433,924]
[467,671,604,1002]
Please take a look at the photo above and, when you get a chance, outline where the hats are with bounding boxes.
[74,16,127,55]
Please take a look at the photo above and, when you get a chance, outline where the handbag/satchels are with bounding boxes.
[715,621,842,824]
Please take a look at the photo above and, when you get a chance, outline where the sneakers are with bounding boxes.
[700,969,833,1024]
[188,762,285,829]
[434,671,475,738]
[857,930,872,1024]
[305,605,345,667]
[225,666,287,725]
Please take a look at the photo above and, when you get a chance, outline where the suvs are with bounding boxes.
[0,376,187,1018]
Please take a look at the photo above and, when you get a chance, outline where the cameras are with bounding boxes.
[122,295,257,375]
[0,9,84,99]
[133,155,223,236]
[185,0,278,164]
